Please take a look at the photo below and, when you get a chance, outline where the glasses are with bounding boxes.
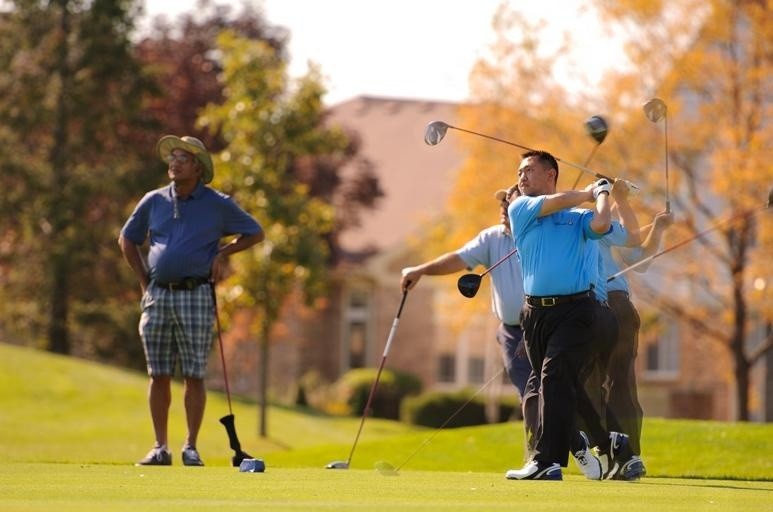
[166,155,194,163]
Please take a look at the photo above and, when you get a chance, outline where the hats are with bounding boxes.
[158,135,213,183]
[497,184,521,202]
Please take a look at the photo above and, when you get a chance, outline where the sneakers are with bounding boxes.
[140,442,172,464]
[182,445,204,466]
[572,430,646,481]
[506,458,562,480]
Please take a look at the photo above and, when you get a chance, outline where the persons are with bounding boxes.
[117,134,265,467]
[400,151,674,482]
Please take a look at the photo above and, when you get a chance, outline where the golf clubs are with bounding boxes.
[644,96,670,213]
[374,355,517,475]
[605,184,773,285]
[424,122,638,191]
[458,249,516,299]
[211,280,255,467]
[572,117,608,191]
[327,281,413,469]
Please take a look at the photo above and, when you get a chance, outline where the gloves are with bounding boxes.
[592,178,610,198]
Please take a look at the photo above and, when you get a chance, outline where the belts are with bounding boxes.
[149,279,211,290]
[524,292,595,309]
